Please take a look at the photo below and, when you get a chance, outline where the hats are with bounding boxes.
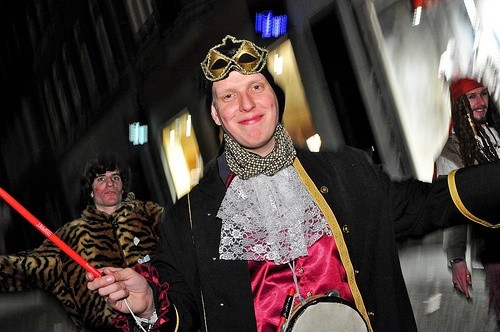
[449,80,482,102]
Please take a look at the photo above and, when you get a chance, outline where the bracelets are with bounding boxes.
[449,258,466,268]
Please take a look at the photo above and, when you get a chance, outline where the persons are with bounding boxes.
[432,77,500,332]
[0,160,165,332]
[86,35,500,331]
